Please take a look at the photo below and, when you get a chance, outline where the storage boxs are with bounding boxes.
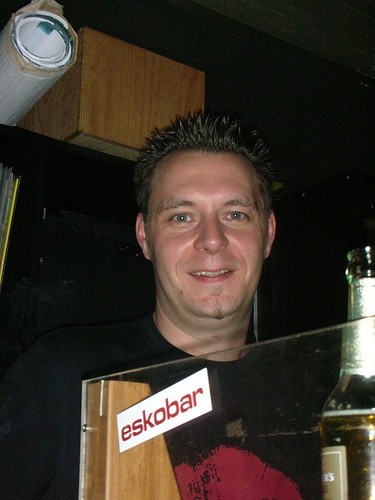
[14,27,205,160]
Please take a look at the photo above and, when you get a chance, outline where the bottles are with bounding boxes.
[317,242,374,500]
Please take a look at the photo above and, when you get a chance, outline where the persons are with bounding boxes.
[0,108,326,500]
